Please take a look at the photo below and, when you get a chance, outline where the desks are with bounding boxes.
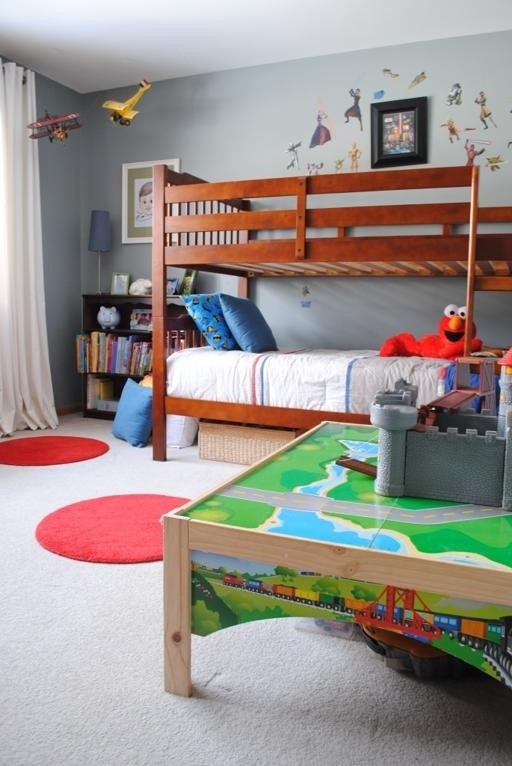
[163,421,512,698]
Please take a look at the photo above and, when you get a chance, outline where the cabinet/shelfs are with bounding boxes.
[80,293,189,421]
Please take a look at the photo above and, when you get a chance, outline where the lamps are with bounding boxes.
[88,210,112,295]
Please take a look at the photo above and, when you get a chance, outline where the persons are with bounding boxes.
[347,142,361,170]
[343,88,365,131]
[441,119,461,141]
[135,182,152,227]
[130,313,152,329]
[463,138,485,164]
[309,111,331,148]
[475,89,499,130]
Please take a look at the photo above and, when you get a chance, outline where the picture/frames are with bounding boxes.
[111,272,130,296]
[121,158,180,246]
[370,96,428,169]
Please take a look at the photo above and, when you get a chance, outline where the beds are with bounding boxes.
[152,165,511,461]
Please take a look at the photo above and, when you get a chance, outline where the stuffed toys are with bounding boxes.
[379,302,483,359]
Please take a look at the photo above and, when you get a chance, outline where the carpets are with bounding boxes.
[34,493,195,564]
[0,435,109,466]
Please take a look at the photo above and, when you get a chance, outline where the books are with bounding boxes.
[74,328,206,411]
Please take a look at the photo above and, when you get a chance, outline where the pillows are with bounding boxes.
[217,292,278,353]
[111,378,157,448]
[179,294,241,351]
